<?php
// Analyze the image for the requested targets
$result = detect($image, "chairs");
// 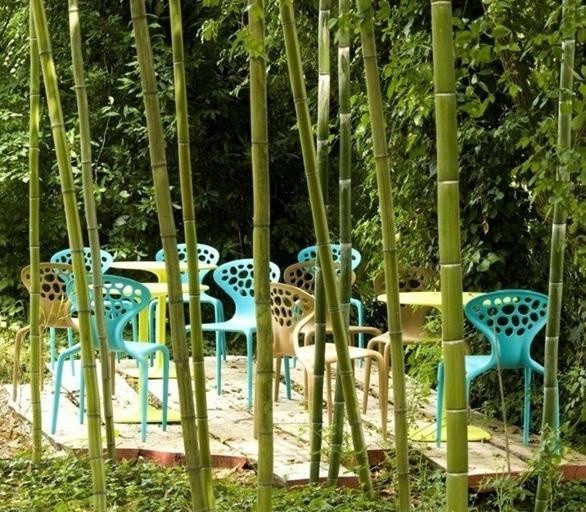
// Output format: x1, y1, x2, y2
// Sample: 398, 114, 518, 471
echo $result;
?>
13, 243, 560, 457
